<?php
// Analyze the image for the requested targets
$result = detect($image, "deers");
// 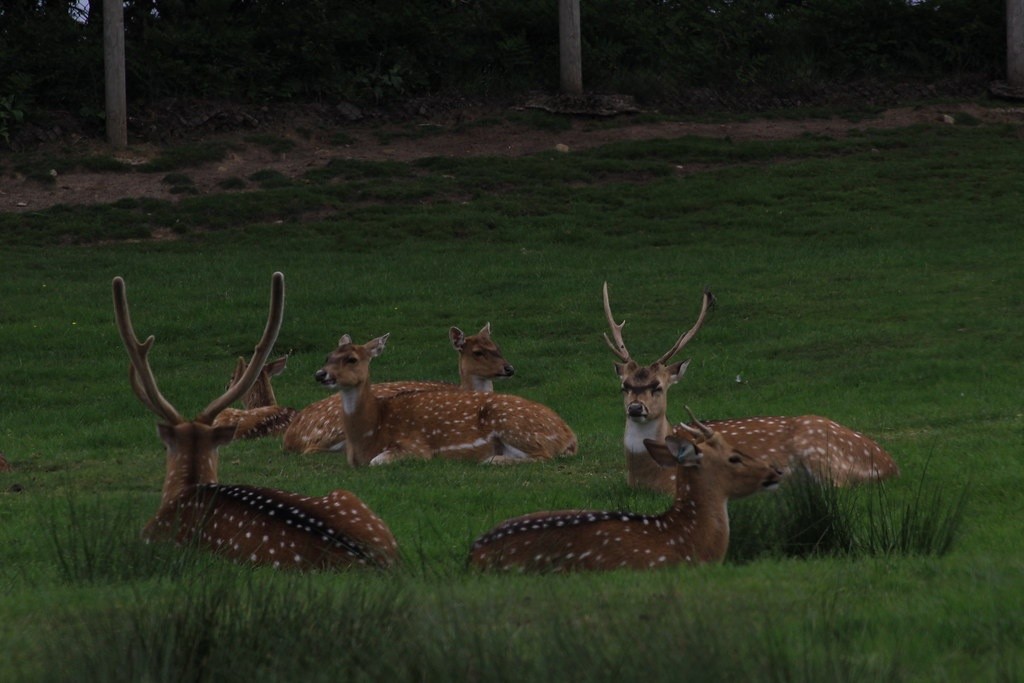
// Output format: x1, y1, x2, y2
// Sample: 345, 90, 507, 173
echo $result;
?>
467, 404, 781, 572
601, 279, 899, 492
112, 270, 399, 575
284, 320, 578, 467
